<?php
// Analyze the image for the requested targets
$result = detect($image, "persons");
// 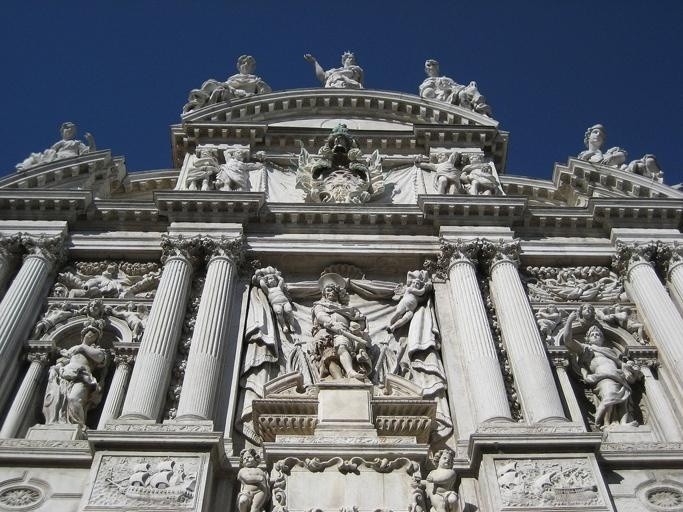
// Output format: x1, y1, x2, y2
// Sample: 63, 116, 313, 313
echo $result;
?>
303, 50, 364, 89
418, 58, 488, 113
461, 154, 499, 196
533, 300, 649, 425
186, 147, 218, 190
15, 121, 97, 176
577, 123, 664, 183
184, 54, 273, 112
214, 145, 264, 189
35, 262, 128, 428
296, 124, 385, 203
417, 148, 464, 194
